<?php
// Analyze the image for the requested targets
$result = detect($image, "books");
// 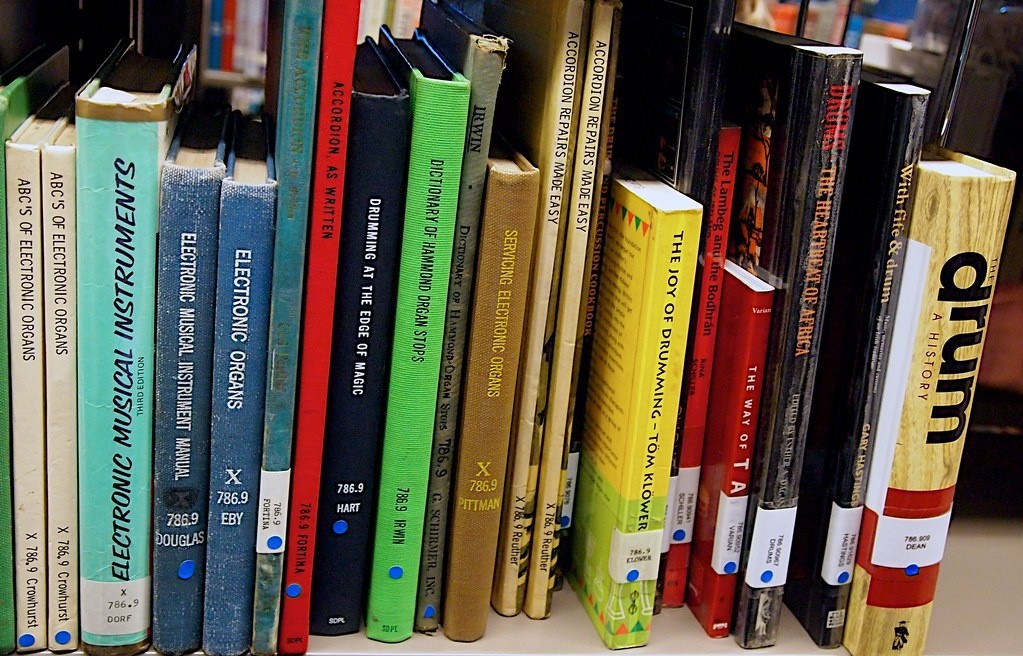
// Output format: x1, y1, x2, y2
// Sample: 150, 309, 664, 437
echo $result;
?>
561, 158, 704, 649
2, 0, 1022, 656
